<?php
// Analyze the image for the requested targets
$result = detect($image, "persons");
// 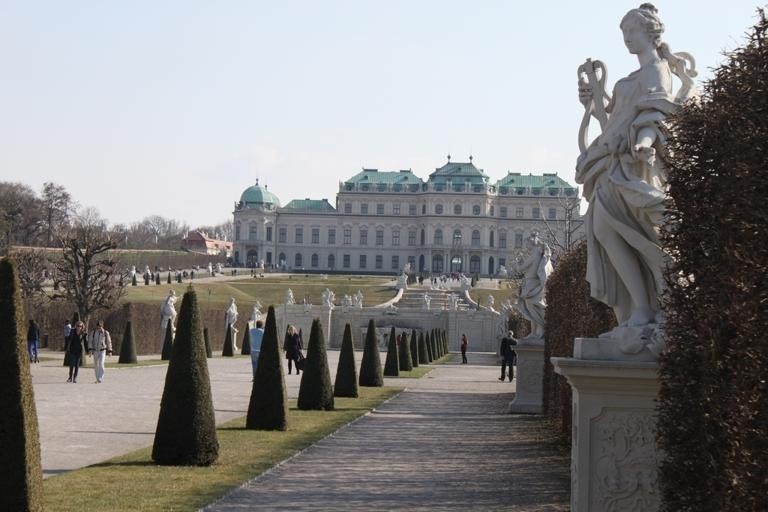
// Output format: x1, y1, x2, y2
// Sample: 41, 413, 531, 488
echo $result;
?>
285, 289, 364, 312
396, 333, 403, 346
498, 330, 518, 382
460, 333, 468, 364
422, 291, 494, 310
419, 271, 468, 285
225, 299, 238, 331
282, 324, 303, 374
87, 318, 113, 383
130, 262, 278, 282
163, 290, 177, 331
26, 319, 40, 363
253, 300, 263, 318
63, 321, 92, 383
60, 320, 72, 351
515, 228, 551, 340
575, 2, 697, 354
248, 320, 265, 381
